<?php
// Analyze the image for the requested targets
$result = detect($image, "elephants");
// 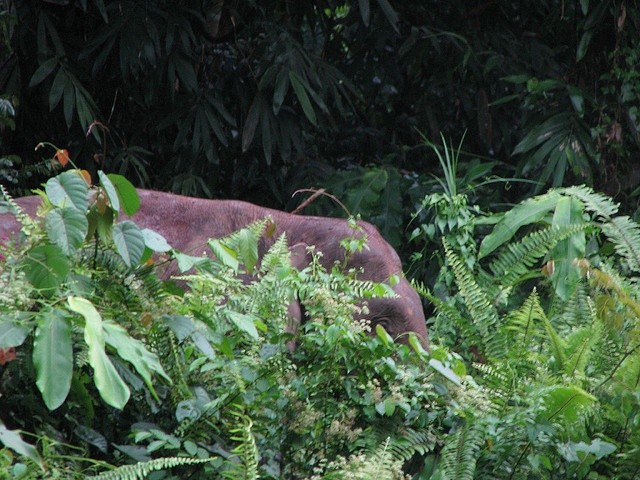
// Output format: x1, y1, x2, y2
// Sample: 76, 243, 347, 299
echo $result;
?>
0, 188, 431, 356
